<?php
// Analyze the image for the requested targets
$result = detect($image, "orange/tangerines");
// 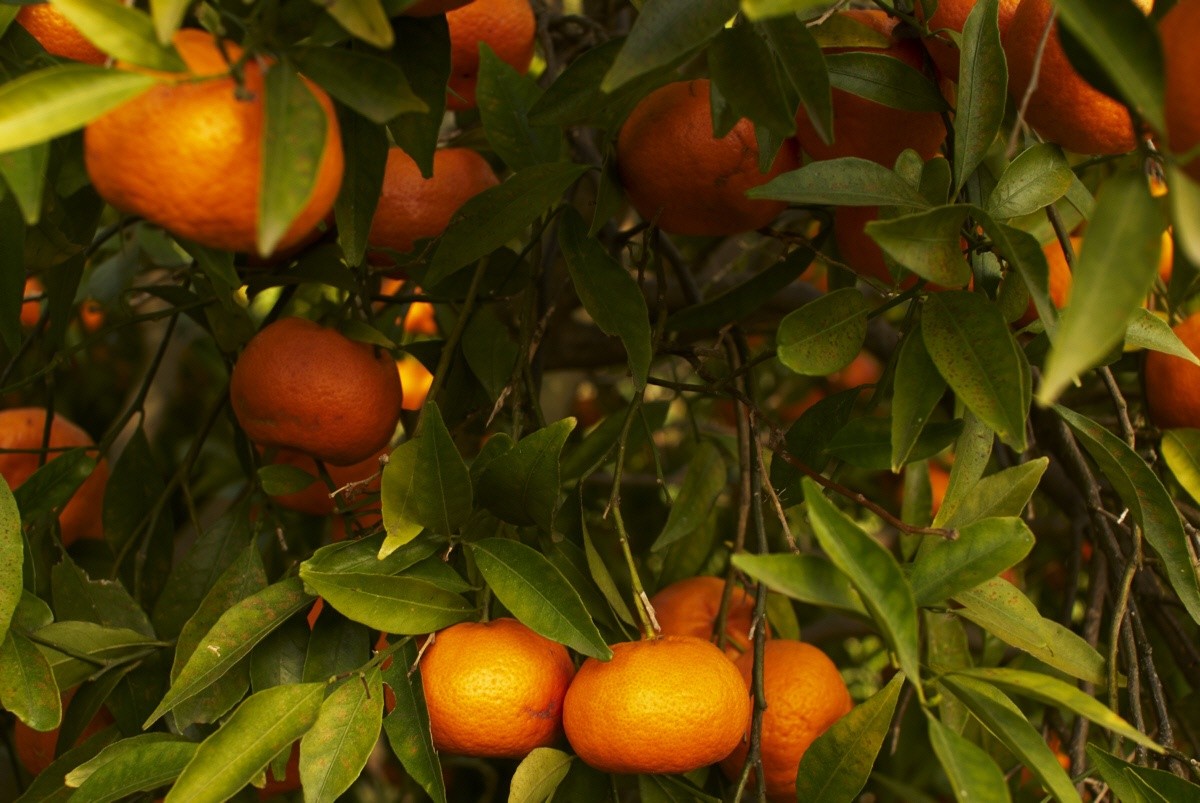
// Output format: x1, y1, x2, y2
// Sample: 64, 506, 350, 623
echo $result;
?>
0, 0, 1199, 802
